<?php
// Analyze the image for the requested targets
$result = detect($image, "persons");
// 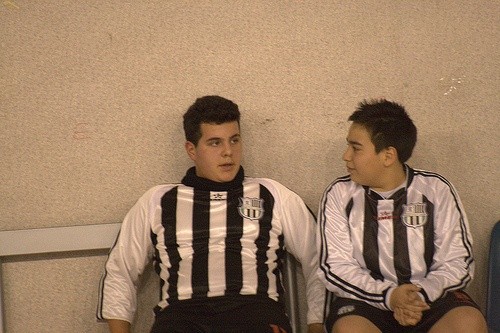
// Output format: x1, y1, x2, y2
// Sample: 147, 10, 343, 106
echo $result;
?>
95, 96, 327, 333
314, 98, 492, 333
